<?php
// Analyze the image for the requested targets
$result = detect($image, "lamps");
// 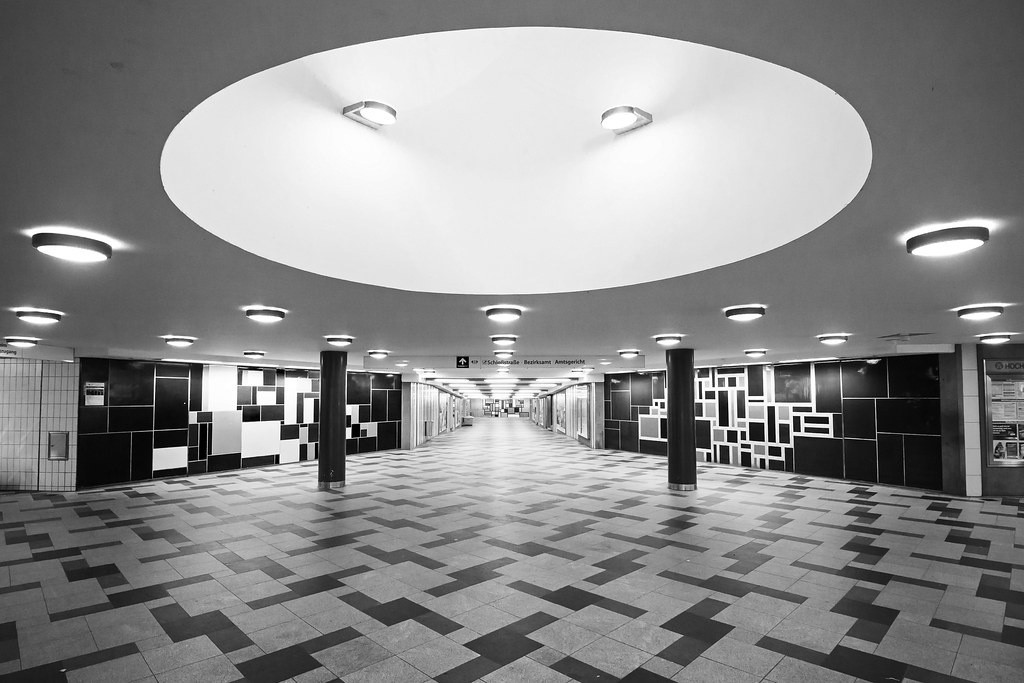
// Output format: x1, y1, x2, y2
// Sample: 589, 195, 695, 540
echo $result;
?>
245, 309, 285, 324
14, 310, 63, 325
979, 335, 1012, 346
165, 337, 193, 348
4, 338, 38, 350
31, 232, 113, 264
325, 337, 353, 348
343, 100, 851, 371
243, 352, 265, 359
957, 306, 1002, 321
905, 226, 989, 259
